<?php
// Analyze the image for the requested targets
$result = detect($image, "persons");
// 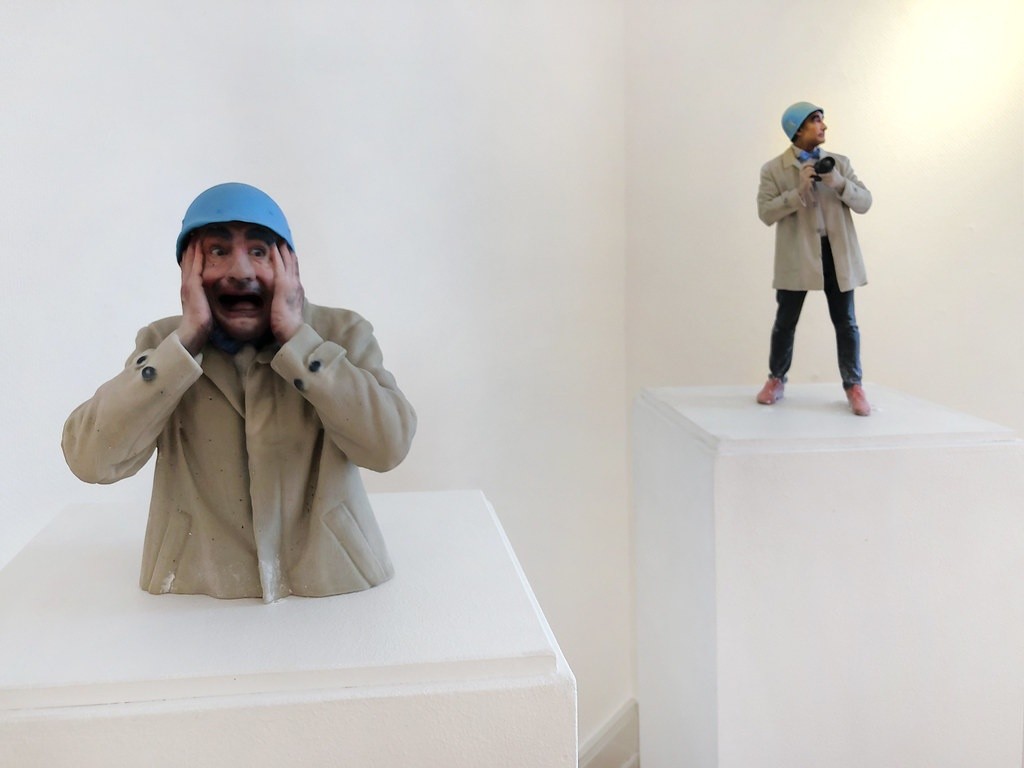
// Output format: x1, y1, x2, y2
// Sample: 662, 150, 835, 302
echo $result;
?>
62, 183, 418, 603
758, 101, 875, 418
